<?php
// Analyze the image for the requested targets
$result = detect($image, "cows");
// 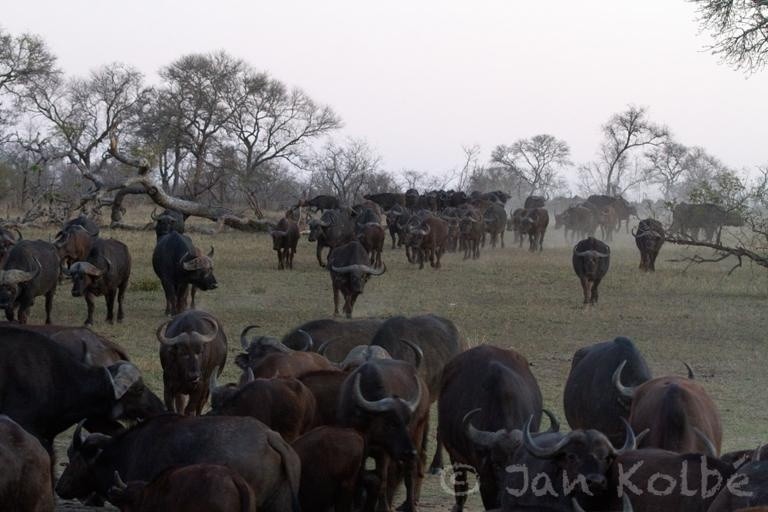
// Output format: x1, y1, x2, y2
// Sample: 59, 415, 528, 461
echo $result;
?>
151, 207, 184, 241
0, 310, 768, 512
1, 214, 132, 327
551, 193, 745, 243
152, 231, 219, 318
630, 217, 664, 274
572, 237, 611, 304
184, 246, 203, 311
327, 240, 388, 319
270, 189, 550, 271
